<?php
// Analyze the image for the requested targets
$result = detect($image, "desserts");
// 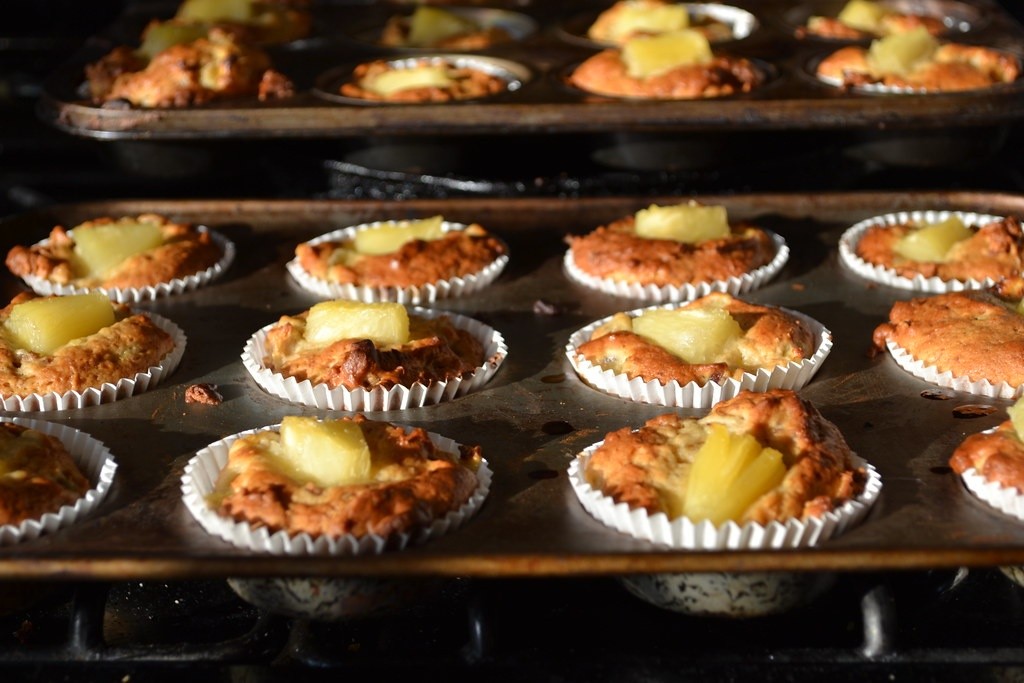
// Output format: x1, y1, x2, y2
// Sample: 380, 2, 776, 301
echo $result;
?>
0, 0, 1024, 551
